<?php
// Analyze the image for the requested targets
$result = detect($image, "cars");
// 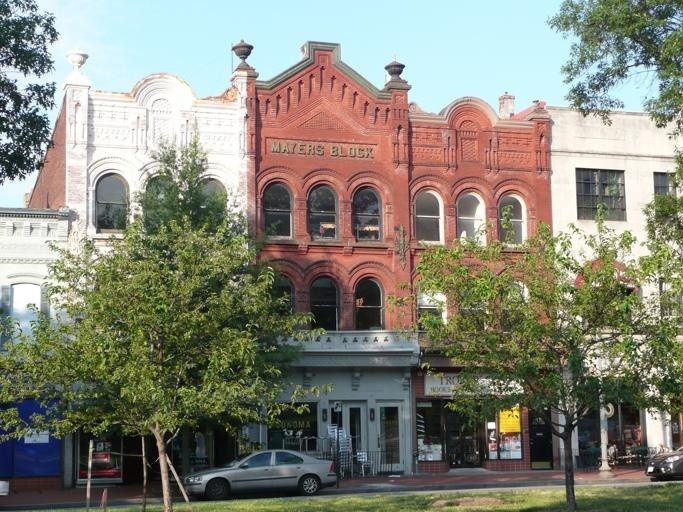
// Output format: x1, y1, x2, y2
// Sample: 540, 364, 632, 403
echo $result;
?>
180, 447, 339, 500
643, 446, 682, 482
